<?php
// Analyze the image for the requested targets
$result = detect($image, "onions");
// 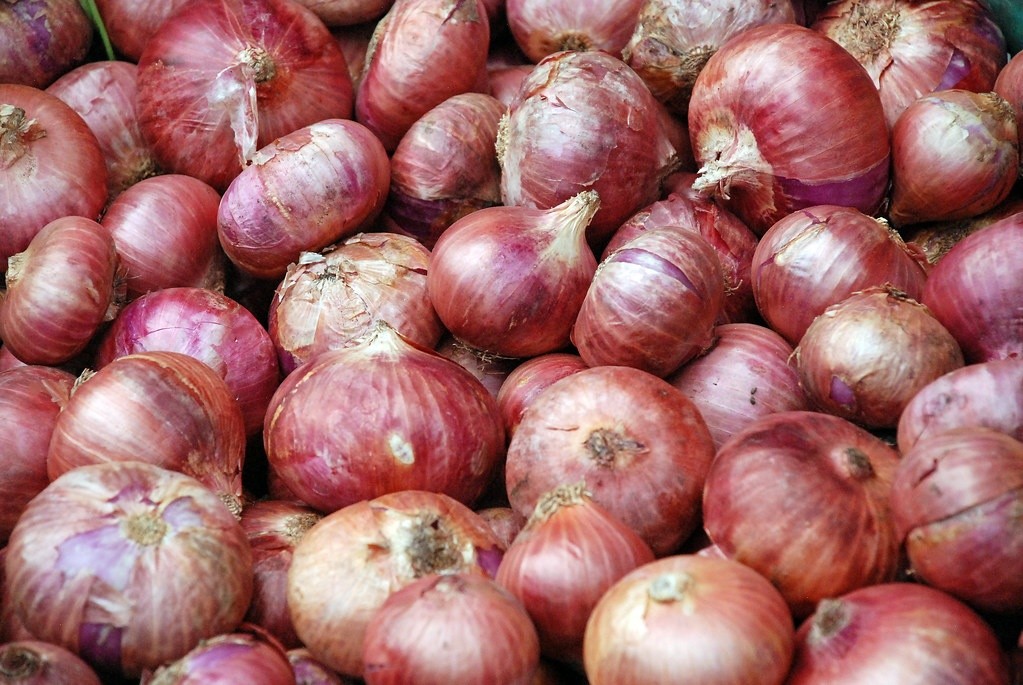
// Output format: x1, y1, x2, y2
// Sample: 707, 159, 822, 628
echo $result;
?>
0, 0, 1023, 685
